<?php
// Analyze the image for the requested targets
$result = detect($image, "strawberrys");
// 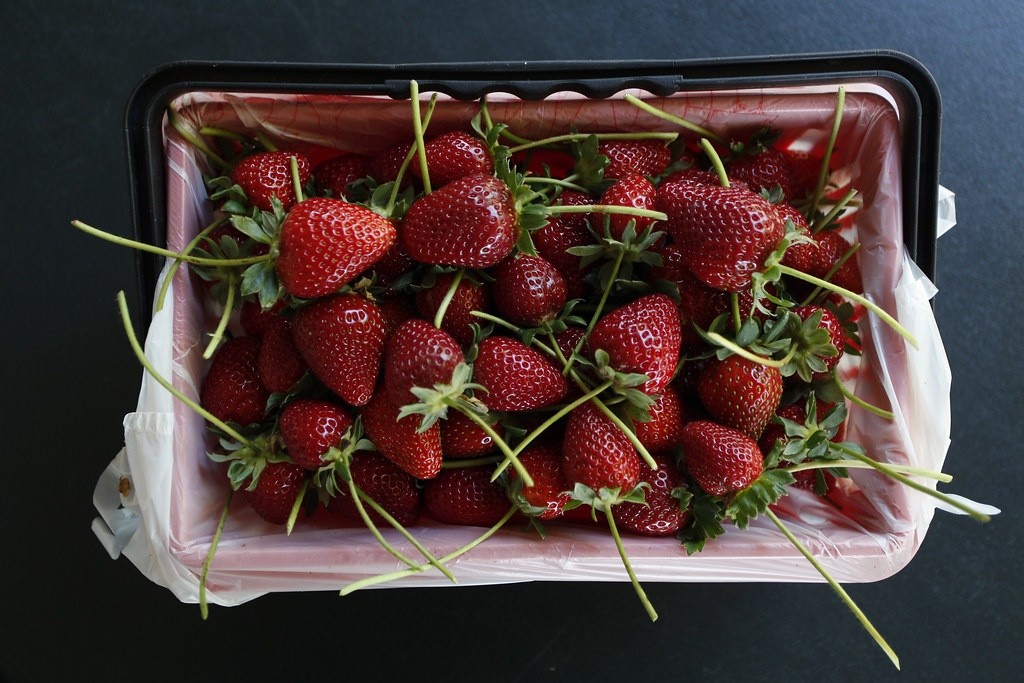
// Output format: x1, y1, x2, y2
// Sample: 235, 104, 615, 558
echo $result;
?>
70, 81, 1001, 672
